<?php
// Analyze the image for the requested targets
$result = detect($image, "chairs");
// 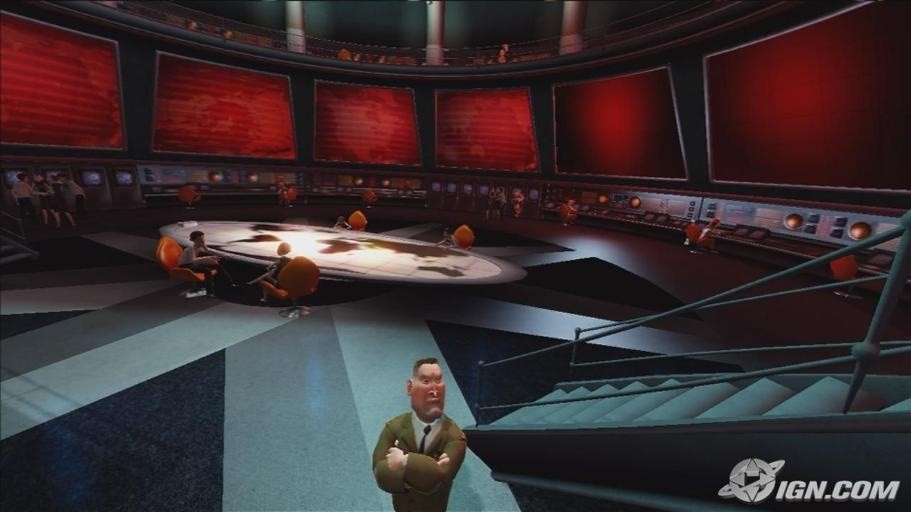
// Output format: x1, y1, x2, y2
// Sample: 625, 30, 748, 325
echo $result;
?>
179, 183, 202, 209
686, 220, 715, 256
280, 184, 298, 208
829, 253, 865, 301
360, 189, 377, 209
